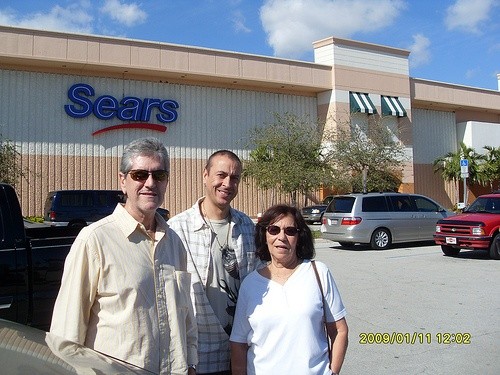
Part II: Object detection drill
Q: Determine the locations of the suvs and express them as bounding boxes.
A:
[43,189,169,227]
[302,195,335,225]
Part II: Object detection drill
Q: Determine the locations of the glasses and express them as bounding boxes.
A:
[129,169,169,181]
[267,225,300,236]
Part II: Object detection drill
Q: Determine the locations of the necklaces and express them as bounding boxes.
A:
[203,204,230,248]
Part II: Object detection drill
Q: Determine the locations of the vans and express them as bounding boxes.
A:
[320,191,459,250]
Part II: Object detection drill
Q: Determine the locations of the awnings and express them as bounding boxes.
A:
[381,96,407,118]
[350,93,377,115]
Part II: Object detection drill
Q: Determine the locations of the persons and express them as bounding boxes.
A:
[229,204,349,375]
[167,150,269,375]
[50,136,200,375]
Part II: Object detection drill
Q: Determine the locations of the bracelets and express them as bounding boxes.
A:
[188,364,196,371]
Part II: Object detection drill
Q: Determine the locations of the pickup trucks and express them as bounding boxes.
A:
[432,193,500,260]
[1,181,81,330]
